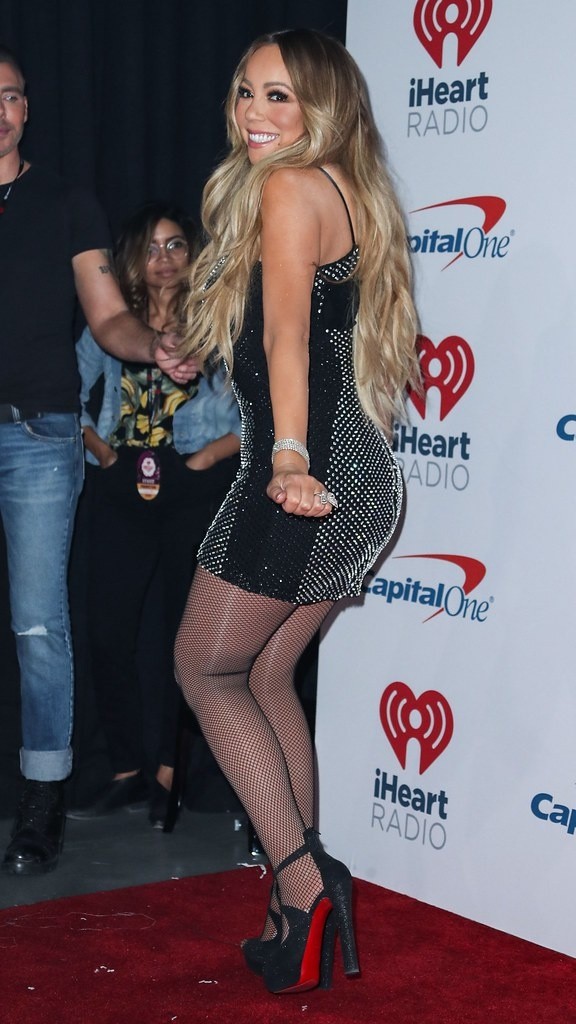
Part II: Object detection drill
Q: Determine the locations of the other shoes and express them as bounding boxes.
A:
[148,774,179,833]
[63,769,152,820]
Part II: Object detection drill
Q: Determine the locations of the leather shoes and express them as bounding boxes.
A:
[2,768,67,875]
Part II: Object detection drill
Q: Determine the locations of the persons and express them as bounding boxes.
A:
[154,26,430,995]
[75,206,246,831]
[0,54,200,878]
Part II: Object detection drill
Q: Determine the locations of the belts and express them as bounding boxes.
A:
[0,401,43,422]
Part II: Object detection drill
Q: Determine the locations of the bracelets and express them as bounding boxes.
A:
[271,439,311,469]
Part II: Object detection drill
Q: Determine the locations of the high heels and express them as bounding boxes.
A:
[261,827,360,993]
[240,876,335,990]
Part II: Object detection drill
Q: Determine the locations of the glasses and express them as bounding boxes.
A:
[145,237,189,265]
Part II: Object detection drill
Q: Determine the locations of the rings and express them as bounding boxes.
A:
[327,491,339,508]
[315,490,327,504]
